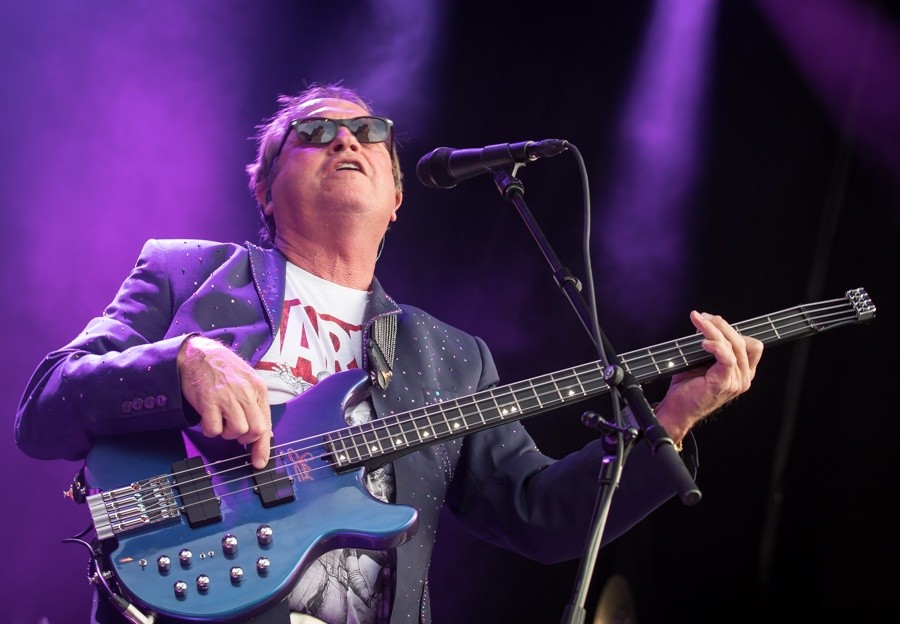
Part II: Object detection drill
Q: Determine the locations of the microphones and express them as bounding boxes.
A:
[416,139,570,188]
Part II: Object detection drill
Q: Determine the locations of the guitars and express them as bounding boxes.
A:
[74,286,877,624]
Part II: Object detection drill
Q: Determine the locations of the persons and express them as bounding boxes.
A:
[14,85,764,624]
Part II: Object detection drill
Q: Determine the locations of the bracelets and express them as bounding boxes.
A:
[651,407,683,451]
